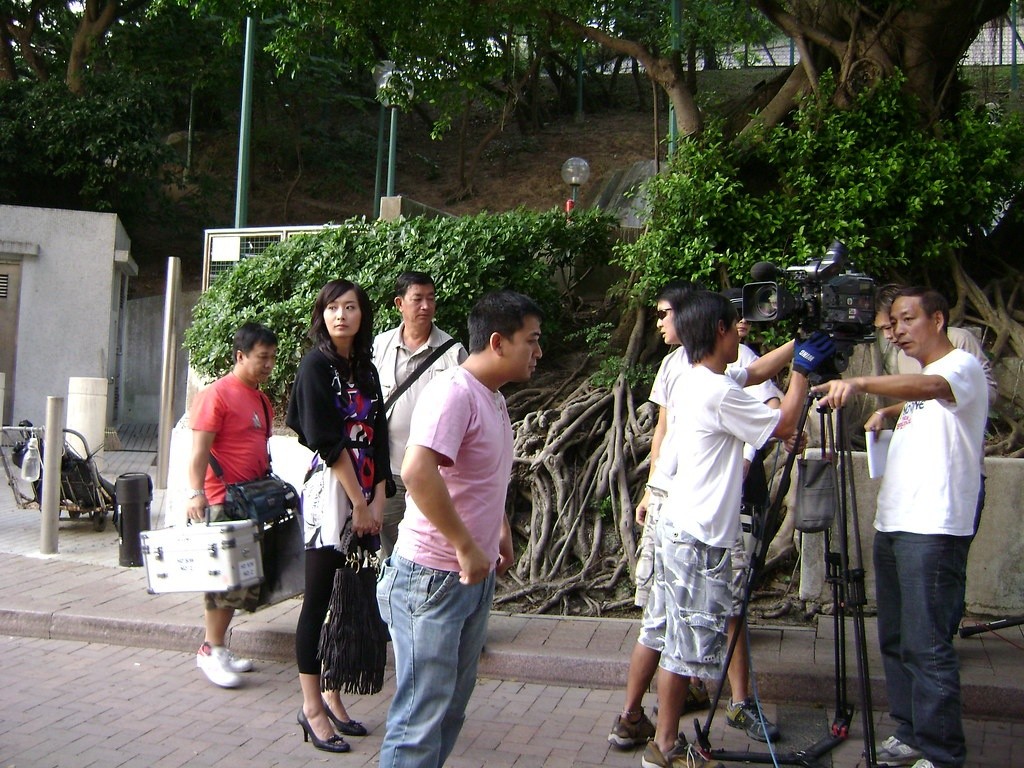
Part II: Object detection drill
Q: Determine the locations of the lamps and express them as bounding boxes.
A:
[560,157,590,207]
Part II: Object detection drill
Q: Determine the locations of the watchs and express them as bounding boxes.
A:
[187,488,205,499]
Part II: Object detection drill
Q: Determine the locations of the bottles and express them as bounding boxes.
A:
[22,439,40,482]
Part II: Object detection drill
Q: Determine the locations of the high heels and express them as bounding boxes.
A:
[297,706,351,753]
[327,707,368,735]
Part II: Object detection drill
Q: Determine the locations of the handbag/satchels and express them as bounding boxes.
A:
[797,459,836,533]
[226,475,302,543]
[318,528,387,695]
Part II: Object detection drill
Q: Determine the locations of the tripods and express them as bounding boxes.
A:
[694,330,890,768]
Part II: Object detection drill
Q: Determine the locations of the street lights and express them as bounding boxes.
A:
[371,60,398,218]
[562,157,592,210]
[376,70,416,195]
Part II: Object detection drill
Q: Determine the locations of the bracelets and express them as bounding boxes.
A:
[875,411,886,420]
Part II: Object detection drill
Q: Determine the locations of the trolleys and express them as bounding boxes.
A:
[0,426,110,533]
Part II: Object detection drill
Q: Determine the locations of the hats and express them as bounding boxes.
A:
[721,287,743,308]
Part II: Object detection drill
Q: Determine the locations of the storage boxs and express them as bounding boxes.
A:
[139,506,266,595]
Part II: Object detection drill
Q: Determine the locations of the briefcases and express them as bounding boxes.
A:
[140,508,267,593]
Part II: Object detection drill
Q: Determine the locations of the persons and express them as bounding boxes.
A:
[811,285,989,768]
[635,279,780,743]
[375,293,544,768]
[369,272,469,559]
[286,278,397,753]
[187,322,279,687]
[874,285,999,633]
[719,288,775,602]
[608,290,837,768]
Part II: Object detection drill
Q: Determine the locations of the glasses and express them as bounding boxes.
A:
[656,309,673,319]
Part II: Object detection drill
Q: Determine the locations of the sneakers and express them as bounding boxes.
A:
[863,736,924,766]
[196,644,240,687]
[654,681,711,715]
[221,647,253,672]
[911,759,935,768]
[609,706,656,747]
[725,695,779,741]
[641,732,724,768]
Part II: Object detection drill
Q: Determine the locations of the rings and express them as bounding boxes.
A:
[375,527,378,531]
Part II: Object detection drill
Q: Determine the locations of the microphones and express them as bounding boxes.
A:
[749,261,790,282]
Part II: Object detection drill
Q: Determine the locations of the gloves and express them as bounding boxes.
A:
[792,330,837,377]
